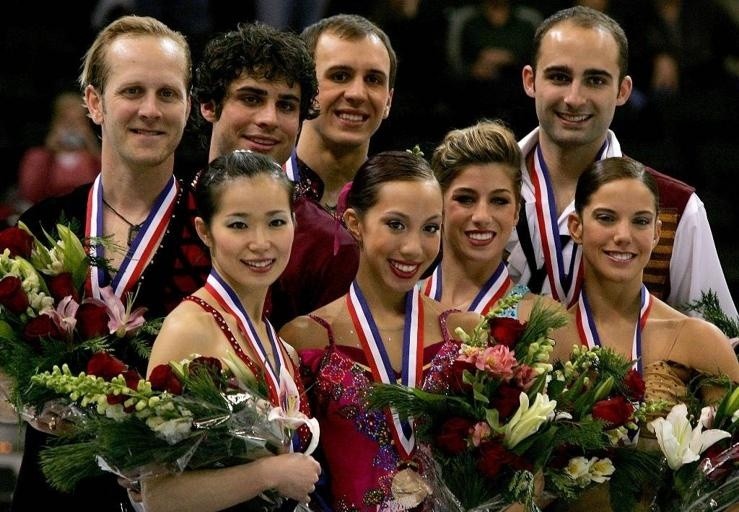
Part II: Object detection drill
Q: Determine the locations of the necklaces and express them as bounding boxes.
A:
[104,197,156,248]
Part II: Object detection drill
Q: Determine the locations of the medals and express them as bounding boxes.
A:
[392,466,429,508]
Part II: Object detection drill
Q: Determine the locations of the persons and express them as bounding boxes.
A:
[278,152,495,512]
[1,14,224,509]
[135,150,321,511]
[419,117,612,511]
[503,6,738,343]
[190,15,359,338]
[1,0,738,186]
[564,157,738,512]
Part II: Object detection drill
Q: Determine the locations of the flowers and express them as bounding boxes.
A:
[0,200,162,433]
[27,355,319,512]
